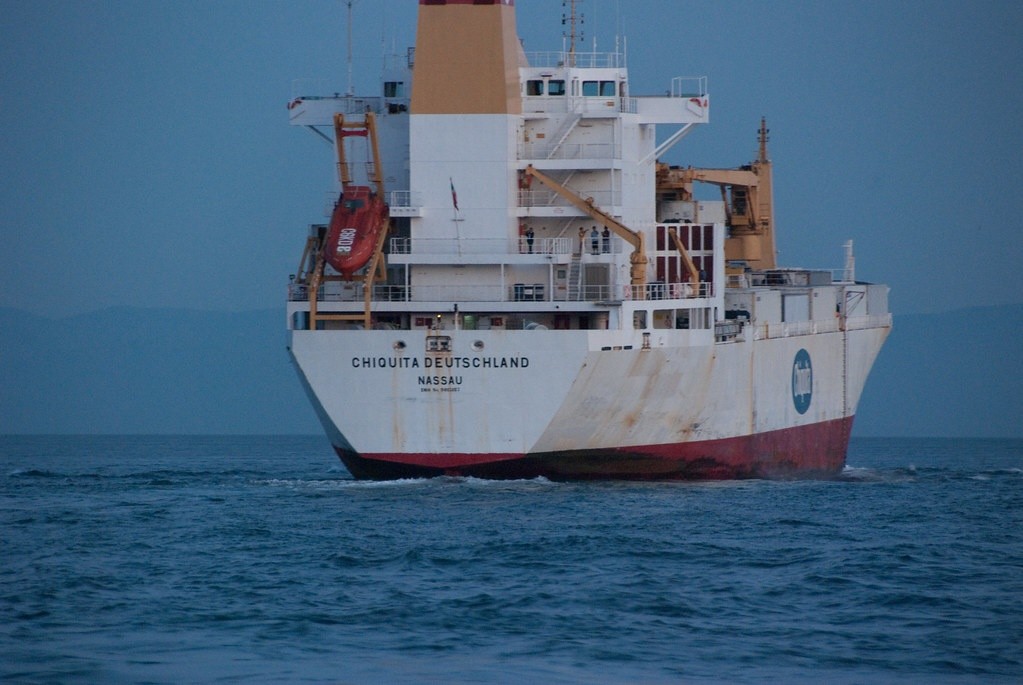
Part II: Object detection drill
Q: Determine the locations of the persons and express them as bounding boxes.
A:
[526,227,534,253]
[578,227,589,252]
[591,226,599,255]
[601,226,610,253]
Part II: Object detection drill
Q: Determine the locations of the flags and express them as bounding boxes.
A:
[450,178,459,211]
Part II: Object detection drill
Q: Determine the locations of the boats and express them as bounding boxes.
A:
[280,0,897,480]
